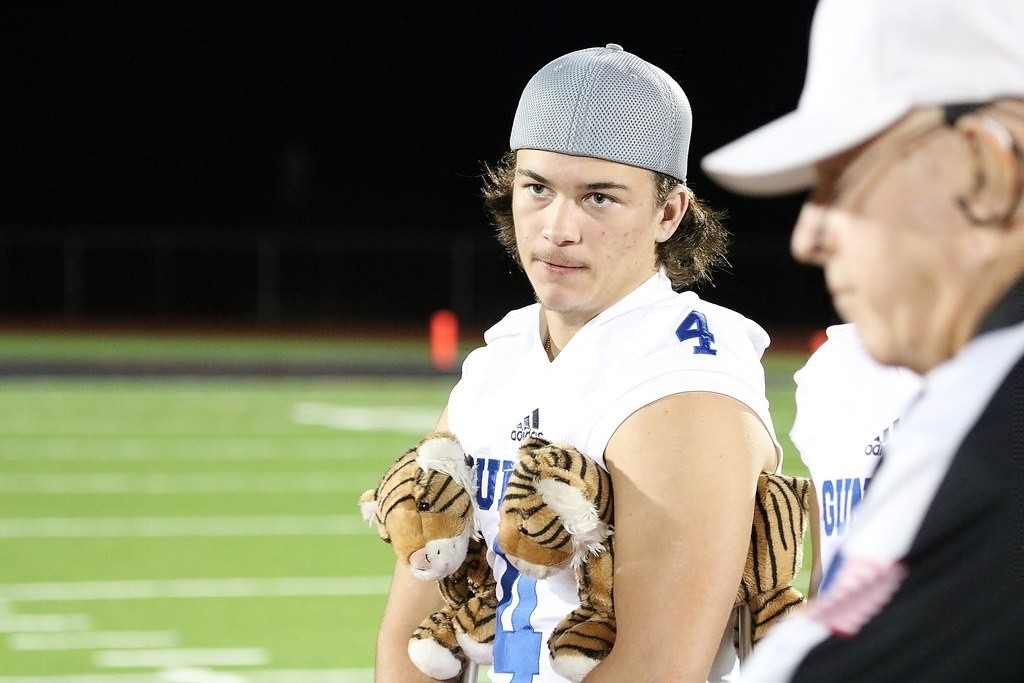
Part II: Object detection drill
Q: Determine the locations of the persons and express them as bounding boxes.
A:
[375,44,784,683]
[700,1,1024,683]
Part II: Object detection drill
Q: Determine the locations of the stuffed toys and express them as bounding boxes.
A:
[498,437,811,683]
[361,432,498,680]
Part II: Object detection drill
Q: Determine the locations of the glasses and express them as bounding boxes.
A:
[815,119,955,210]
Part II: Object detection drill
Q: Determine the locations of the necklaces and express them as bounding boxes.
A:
[545,332,552,354]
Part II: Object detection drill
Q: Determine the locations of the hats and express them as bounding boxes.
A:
[510,43,692,182]
[699,0,1024,196]
